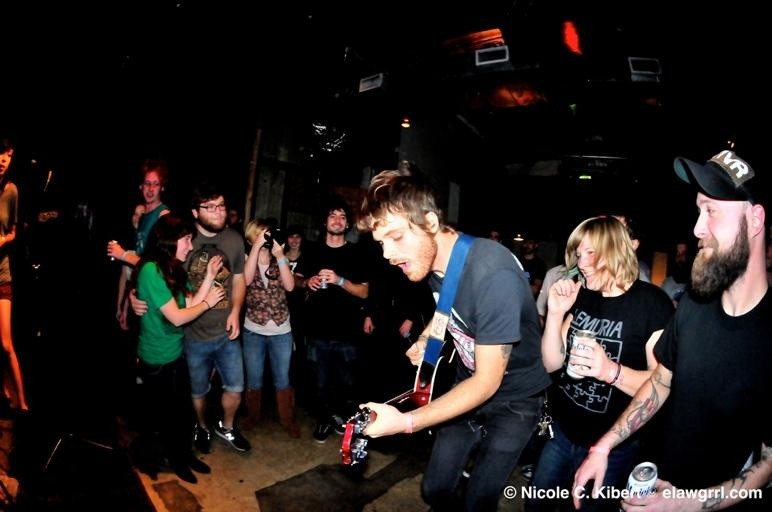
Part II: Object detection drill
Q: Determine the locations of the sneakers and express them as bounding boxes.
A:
[172,389,346,483]
[520,464,533,481]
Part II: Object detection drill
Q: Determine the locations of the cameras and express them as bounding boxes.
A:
[264,228,286,248]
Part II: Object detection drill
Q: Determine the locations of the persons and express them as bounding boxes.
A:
[1,143,692,454]
[524,214,676,511]
[569,140,772,512]
[358,168,549,509]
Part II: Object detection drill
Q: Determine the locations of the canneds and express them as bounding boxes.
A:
[320,278,328,289]
[566,329,595,380]
[626,461,658,498]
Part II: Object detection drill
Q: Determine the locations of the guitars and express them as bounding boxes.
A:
[339,340,458,466]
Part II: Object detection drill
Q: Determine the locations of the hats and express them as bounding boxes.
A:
[673,146,770,201]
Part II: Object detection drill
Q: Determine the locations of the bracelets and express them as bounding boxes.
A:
[602,358,626,385]
[416,334,428,342]
[403,408,416,434]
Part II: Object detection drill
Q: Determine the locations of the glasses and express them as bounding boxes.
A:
[201,203,225,212]
